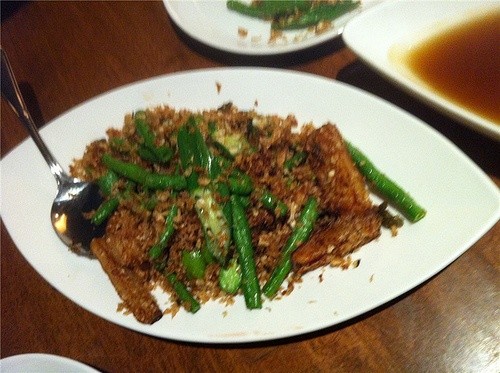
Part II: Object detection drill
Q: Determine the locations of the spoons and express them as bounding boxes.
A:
[1,51,106,260]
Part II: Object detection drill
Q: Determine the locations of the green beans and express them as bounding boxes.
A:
[91,110,427,313]
[227,0,359,30]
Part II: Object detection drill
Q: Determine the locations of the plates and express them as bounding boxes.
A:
[338,3,499,142]
[0,69,500,347]
[0,354,98,373]
[154,1,374,60]
